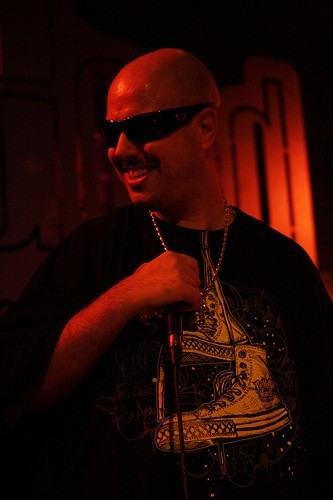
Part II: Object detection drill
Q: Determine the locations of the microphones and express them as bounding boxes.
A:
[130,222,185,366]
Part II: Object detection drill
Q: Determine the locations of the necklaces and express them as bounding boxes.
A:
[147,198,231,297]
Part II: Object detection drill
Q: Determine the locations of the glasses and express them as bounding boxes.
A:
[100,104,213,146]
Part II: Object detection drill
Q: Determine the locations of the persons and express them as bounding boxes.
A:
[1,47,333,500]
